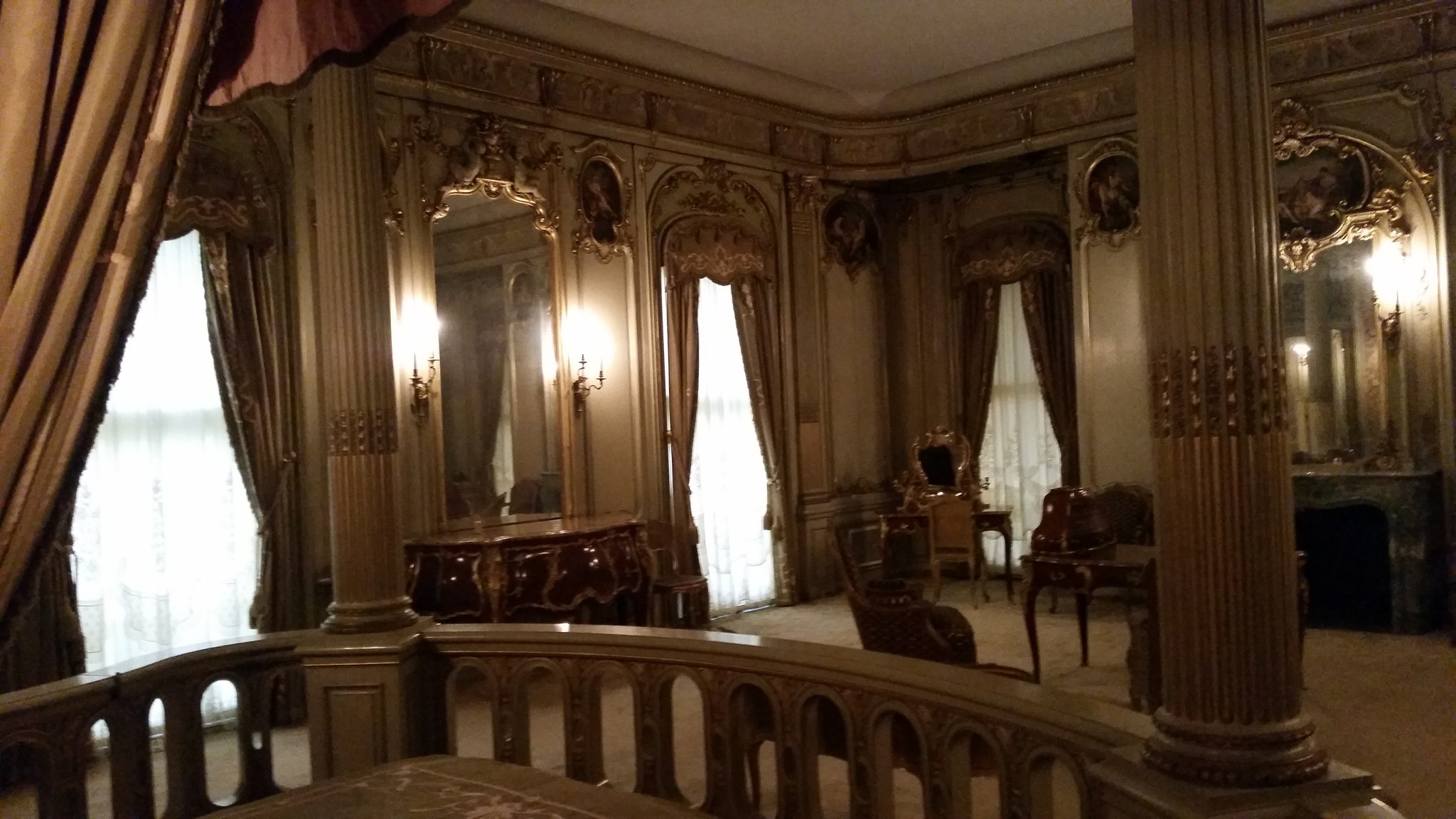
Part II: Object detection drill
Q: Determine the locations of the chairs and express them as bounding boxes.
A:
[927,500,991,610]
[1048,481,1155,615]
[837,555,1031,681]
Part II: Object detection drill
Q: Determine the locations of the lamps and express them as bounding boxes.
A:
[1367,253,1404,356]
[400,315,440,426]
[566,312,608,418]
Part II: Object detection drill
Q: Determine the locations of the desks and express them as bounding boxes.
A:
[1017,543,1309,712]
[880,504,1018,608]
[403,514,659,627]
[1290,467,1443,633]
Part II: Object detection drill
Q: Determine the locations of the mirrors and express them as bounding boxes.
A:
[911,427,969,497]
[1273,211,1391,462]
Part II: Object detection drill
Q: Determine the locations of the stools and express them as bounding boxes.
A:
[653,574,710,631]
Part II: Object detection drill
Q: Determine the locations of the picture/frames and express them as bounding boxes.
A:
[566,138,639,264]
[1272,140,1371,239]
[822,185,884,283]
[1072,136,1144,256]
